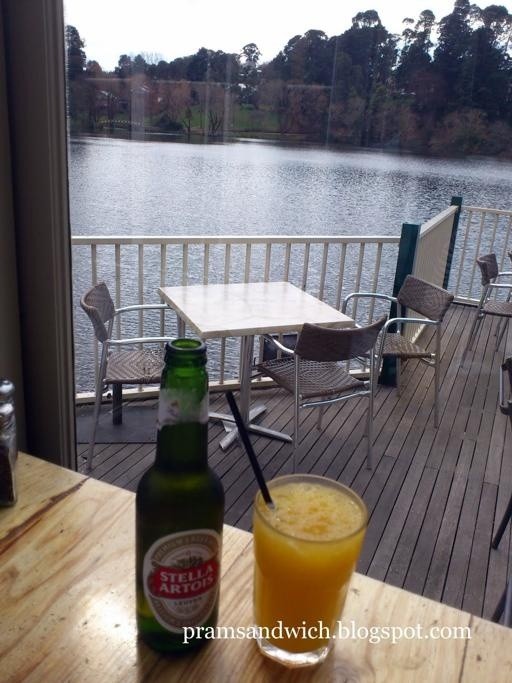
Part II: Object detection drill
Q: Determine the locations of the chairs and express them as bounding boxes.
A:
[459,251,512,368]
[80,280,182,470]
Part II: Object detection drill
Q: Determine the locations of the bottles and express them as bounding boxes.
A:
[135,337,225,655]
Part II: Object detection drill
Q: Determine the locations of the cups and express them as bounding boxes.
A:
[252,473,368,669]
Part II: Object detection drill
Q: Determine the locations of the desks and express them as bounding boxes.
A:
[156,279,357,452]
[1,451,511,683]
